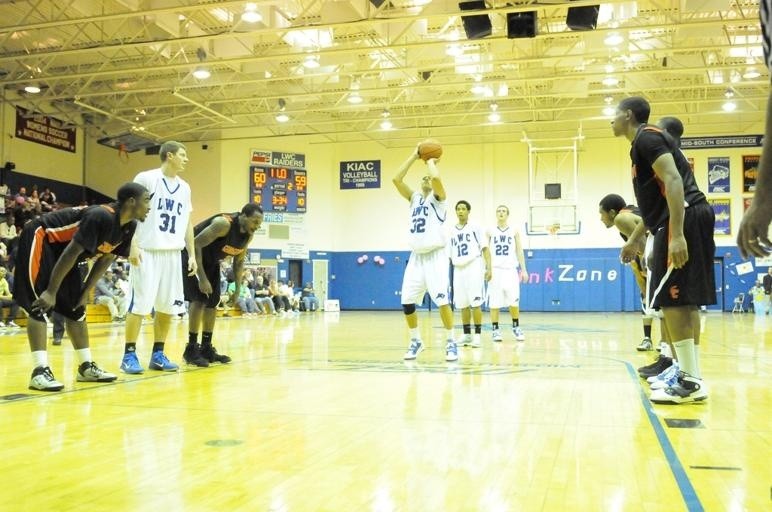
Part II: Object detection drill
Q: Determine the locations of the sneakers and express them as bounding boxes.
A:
[404,338,424,359]
[464,334,480,348]
[119,352,144,374]
[512,327,525,341]
[637,337,708,405]
[149,351,179,371]
[29,366,64,392]
[445,339,459,360]
[0,321,6,327]
[241,308,321,316]
[76,362,118,381]
[183,343,231,367]
[493,329,503,341]
[7,320,17,326]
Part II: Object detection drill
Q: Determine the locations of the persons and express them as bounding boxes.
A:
[487,206,527,343]
[599,95,714,404]
[748,280,766,316]
[11,182,152,391]
[214,253,324,318]
[120,141,198,375]
[0,184,191,330]
[762,266,772,316]
[391,143,460,362]
[182,202,263,368]
[448,201,493,347]
[735,0,772,260]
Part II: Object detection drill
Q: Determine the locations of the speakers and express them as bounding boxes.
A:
[459,1,493,38]
[507,1,538,38]
[566,0,599,29]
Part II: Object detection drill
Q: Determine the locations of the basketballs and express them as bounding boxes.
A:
[419,138,442,162]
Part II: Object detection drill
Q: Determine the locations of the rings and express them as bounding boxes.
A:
[748,239,757,243]
